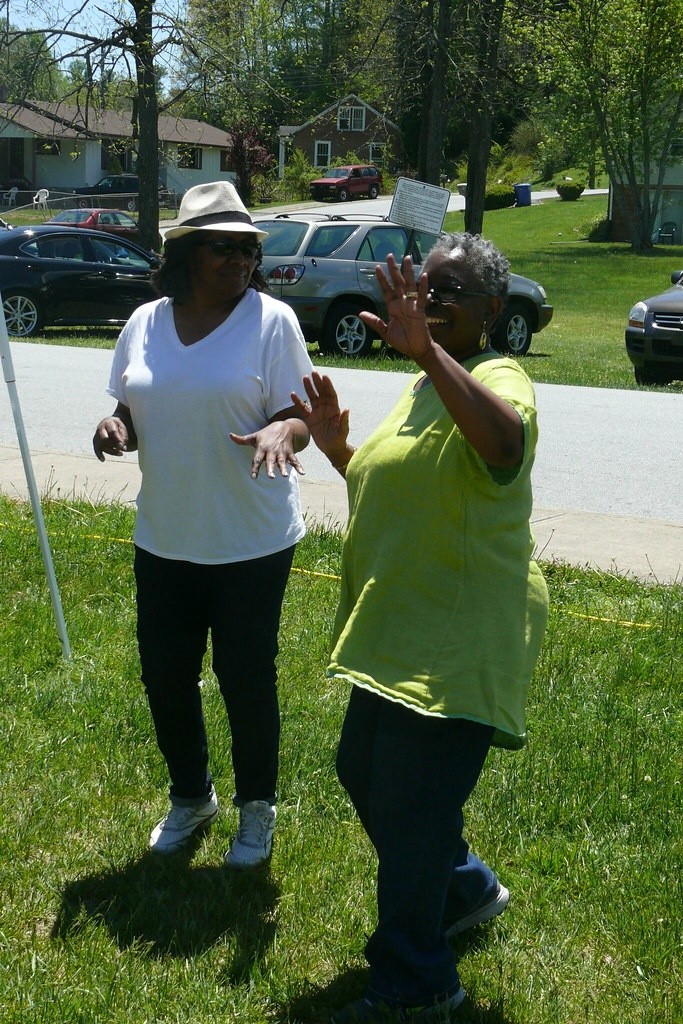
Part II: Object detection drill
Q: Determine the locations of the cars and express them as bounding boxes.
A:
[0,209,165,337]
[625,272,683,386]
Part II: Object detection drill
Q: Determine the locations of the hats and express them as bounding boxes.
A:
[165,181,269,245]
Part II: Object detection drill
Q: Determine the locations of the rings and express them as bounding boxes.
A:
[406,292,419,297]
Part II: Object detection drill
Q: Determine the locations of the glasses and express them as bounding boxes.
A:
[417,283,490,303]
[185,237,260,257]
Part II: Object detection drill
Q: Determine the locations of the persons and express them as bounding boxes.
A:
[92,179,313,863]
[290,229,548,1016]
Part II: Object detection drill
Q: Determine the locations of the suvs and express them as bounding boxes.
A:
[72,173,170,212]
[310,166,383,202]
[251,213,554,357]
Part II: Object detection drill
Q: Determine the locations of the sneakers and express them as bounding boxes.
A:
[226,799,278,868]
[150,785,218,852]
[330,972,464,1024]
[441,878,509,938]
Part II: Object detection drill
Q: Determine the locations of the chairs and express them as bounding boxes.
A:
[657,221,677,243]
[374,243,402,263]
[2,187,18,208]
[32,189,49,210]
[102,217,110,224]
[63,242,75,260]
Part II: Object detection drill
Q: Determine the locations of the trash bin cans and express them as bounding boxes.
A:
[514,183,531,206]
